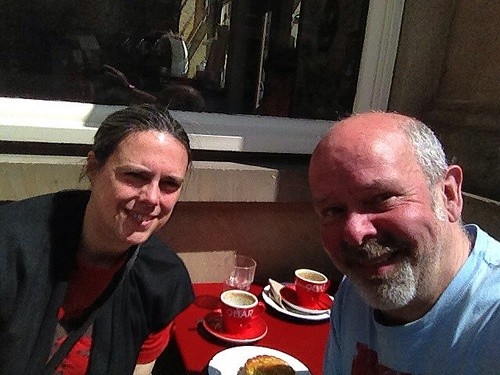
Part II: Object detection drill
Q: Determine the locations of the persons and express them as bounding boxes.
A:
[307,111,500,375]
[0,102,194,374]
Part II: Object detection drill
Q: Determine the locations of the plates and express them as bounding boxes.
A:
[207,344,312,375]
[261,282,336,321]
[202,309,268,345]
[279,282,335,314]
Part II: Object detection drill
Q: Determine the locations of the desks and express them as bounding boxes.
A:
[173,282,333,375]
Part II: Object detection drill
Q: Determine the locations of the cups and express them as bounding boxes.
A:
[223,254,257,295]
[294,268,328,307]
[220,289,266,335]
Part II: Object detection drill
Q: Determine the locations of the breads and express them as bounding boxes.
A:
[245,355,296,375]
